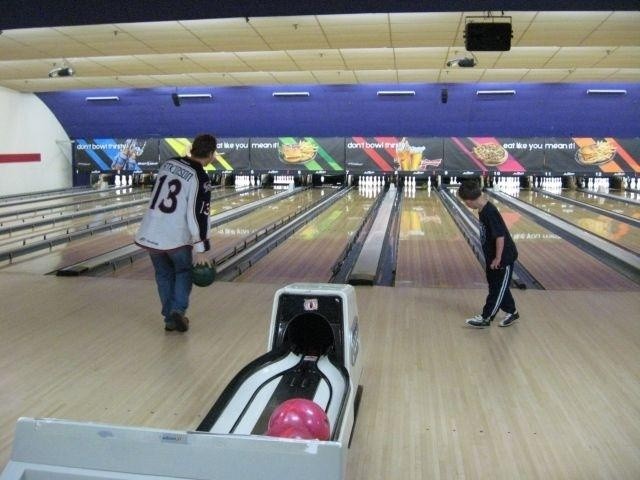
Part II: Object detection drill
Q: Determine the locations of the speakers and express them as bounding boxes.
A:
[172,93,179,106]
[442,89,447,103]
[465,23,511,51]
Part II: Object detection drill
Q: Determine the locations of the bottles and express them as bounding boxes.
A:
[113,147,137,172]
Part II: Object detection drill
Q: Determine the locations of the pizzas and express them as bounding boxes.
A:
[472,143,506,164]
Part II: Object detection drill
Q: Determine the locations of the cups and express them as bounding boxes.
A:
[395,146,424,171]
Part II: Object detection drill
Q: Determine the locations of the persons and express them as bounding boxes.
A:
[133,134,217,332]
[458,179,519,328]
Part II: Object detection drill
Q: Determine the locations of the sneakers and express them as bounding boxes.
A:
[497,310,520,328]
[164,313,189,332]
[465,315,491,327]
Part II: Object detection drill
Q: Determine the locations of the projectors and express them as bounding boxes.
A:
[48,66,73,77]
[447,58,474,67]
